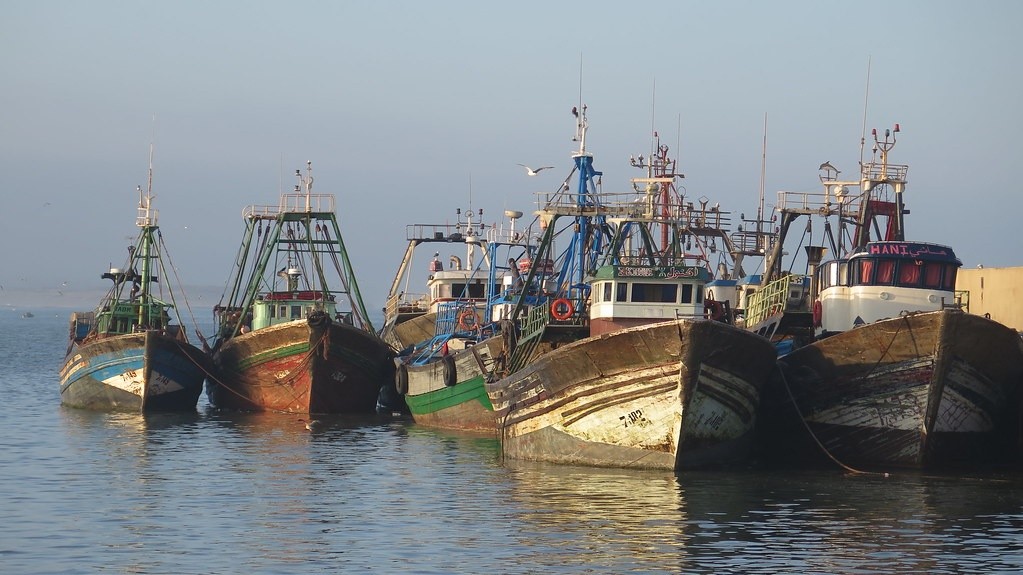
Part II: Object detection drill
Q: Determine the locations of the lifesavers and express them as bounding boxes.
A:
[812,301,821,327]
[231,312,248,329]
[395,364,408,394]
[705,300,723,320]
[460,309,479,331]
[552,298,573,319]
[443,355,456,386]
[306,310,332,330]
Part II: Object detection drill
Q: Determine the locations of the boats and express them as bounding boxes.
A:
[380,51,1023,475]
[57,117,212,413]
[205,146,397,418]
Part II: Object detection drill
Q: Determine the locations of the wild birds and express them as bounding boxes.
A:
[517,164,554,176]
[564,182,569,192]
